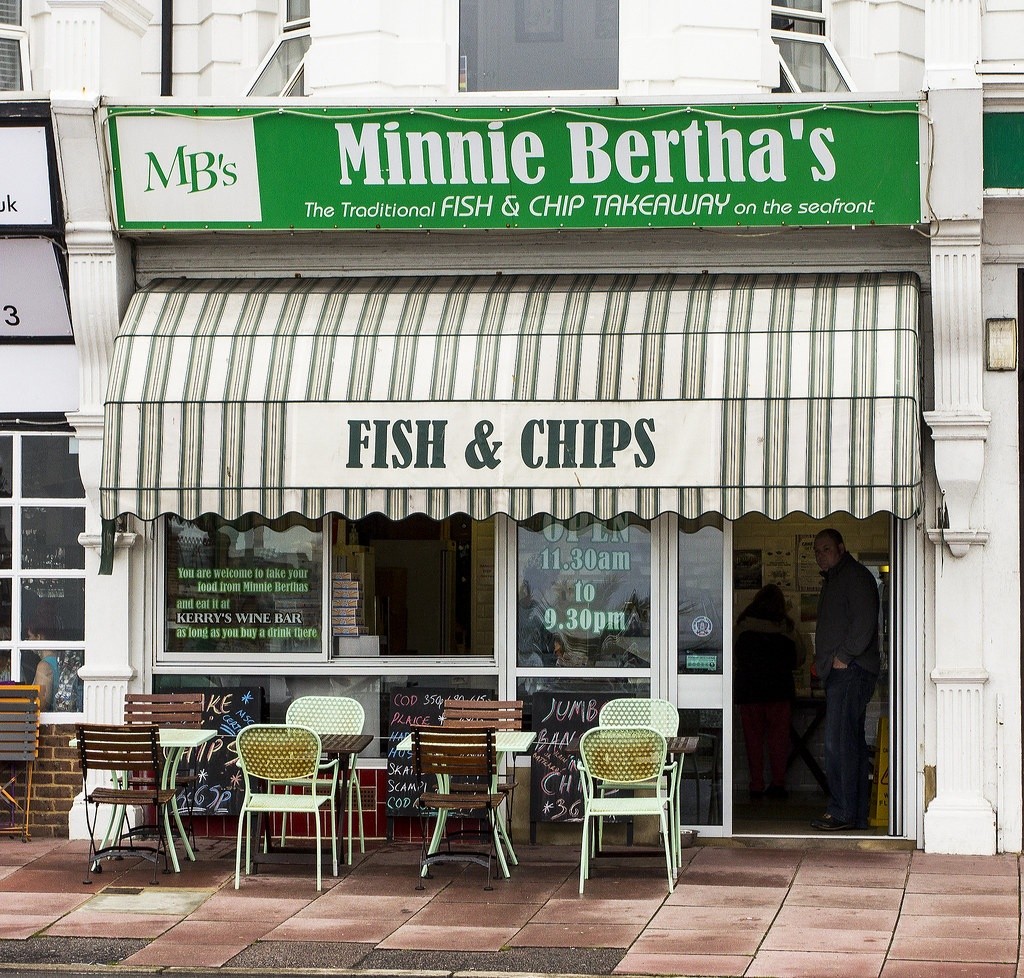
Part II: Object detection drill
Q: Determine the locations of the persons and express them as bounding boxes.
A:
[808,528,880,832]
[0,649,26,685]
[28,618,81,710]
[732,584,808,792]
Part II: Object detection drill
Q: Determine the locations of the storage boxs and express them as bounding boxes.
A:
[332,572,369,636]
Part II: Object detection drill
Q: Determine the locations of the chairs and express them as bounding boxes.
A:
[577,699,684,894]
[410,699,523,891]
[235,696,365,891]
[0,682,41,842]
[74,693,202,884]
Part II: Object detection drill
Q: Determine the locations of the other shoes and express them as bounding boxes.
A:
[749,790,768,802]
[810,810,868,831]
[766,782,792,796]
[814,795,833,812]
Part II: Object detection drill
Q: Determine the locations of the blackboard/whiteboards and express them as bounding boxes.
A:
[156,686,266,817]
[389,686,495,818]
[530,691,634,823]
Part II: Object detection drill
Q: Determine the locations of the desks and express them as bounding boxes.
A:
[774,696,827,791]
[564,736,700,881]
[396,731,537,878]
[251,734,374,875]
[69,728,218,873]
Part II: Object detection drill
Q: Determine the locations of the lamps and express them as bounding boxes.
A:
[986,315,1017,372]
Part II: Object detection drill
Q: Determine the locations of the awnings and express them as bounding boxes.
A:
[97,276,930,520]
